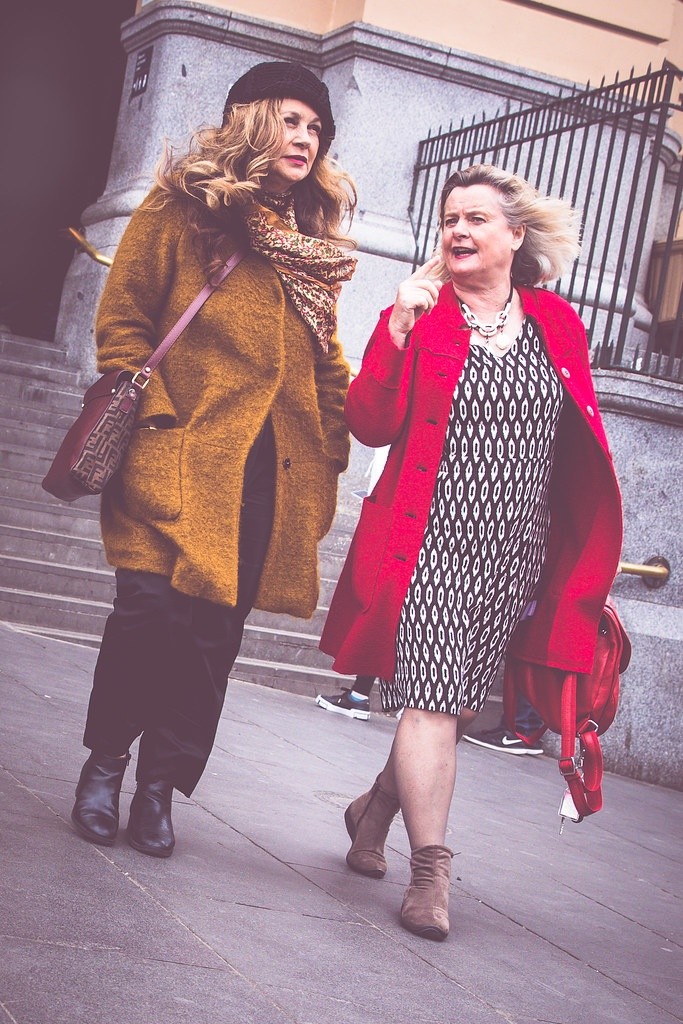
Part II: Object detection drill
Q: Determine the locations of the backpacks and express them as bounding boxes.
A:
[502,591,632,818]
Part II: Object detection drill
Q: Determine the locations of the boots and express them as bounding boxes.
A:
[70,750,131,846]
[127,763,175,858]
[342,771,401,879]
[400,843,463,940]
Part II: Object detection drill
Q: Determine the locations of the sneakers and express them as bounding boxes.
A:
[316,685,371,720]
[464,725,544,755]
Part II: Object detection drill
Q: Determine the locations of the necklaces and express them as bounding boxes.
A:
[455,277,514,350]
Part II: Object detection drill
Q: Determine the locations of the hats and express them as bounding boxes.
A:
[224,61,337,159]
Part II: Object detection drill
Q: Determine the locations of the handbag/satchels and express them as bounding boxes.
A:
[43,370,142,501]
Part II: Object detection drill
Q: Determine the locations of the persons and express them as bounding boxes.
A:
[343,165,624,942]
[71,62,351,858]
[314,675,376,721]
[461,687,544,755]
